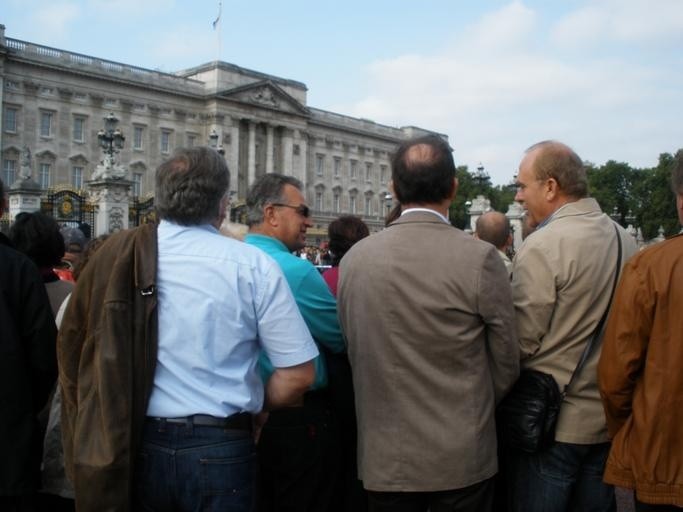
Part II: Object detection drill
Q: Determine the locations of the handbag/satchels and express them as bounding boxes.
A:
[492,366,565,460]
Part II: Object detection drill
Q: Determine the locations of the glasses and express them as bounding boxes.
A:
[270,199,310,219]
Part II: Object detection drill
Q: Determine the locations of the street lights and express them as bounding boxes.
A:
[471,162,491,197]
[204,128,225,157]
[463,198,471,230]
[382,190,391,224]
[624,208,636,226]
[87,112,132,243]
[609,205,622,224]
[511,172,519,200]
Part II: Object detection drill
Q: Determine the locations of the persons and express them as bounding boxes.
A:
[12,210,546,330]
[597,146,682,512]
[54,143,321,512]
[239,172,345,512]
[509,138,642,512]
[334,127,520,511]
[0,175,60,512]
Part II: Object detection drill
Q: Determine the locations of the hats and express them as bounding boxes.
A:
[59,224,86,254]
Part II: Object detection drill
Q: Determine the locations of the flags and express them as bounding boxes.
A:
[211,8,221,30]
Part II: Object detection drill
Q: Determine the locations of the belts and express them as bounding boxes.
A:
[144,414,257,431]
